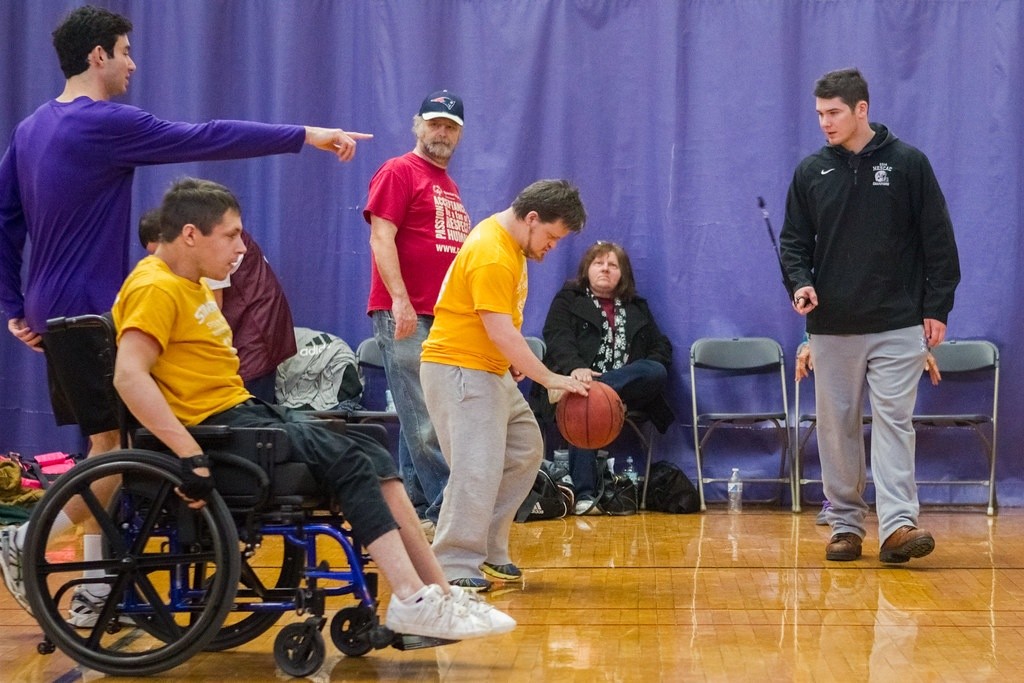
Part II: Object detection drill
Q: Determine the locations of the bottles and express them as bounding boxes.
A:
[621,456,638,486]
[727,468,742,514]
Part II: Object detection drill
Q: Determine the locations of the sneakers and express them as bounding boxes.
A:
[69,585,137,627]
[0,525,34,617]
[879,525,935,563]
[450,577,491,591]
[479,562,521,580]
[825,533,862,561]
[385,584,491,640]
[450,588,516,634]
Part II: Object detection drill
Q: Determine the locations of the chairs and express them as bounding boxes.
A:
[356,337,1001,514]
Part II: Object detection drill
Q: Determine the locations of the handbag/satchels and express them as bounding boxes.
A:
[572,460,637,516]
[647,460,700,514]
[513,459,575,523]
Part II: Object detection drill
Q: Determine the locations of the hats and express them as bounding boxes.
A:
[419,90,464,126]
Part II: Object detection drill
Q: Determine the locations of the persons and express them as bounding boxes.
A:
[416,178,592,594]
[136,206,299,402]
[535,237,673,515]
[0,5,375,625]
[109,181,518,641]
[363,89,473,539]
[777,69,963,565]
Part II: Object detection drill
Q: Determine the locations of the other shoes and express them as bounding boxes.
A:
[547,390,564,405]
[571,499,602,516]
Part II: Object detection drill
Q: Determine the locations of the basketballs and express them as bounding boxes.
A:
[556,379,625,449]
